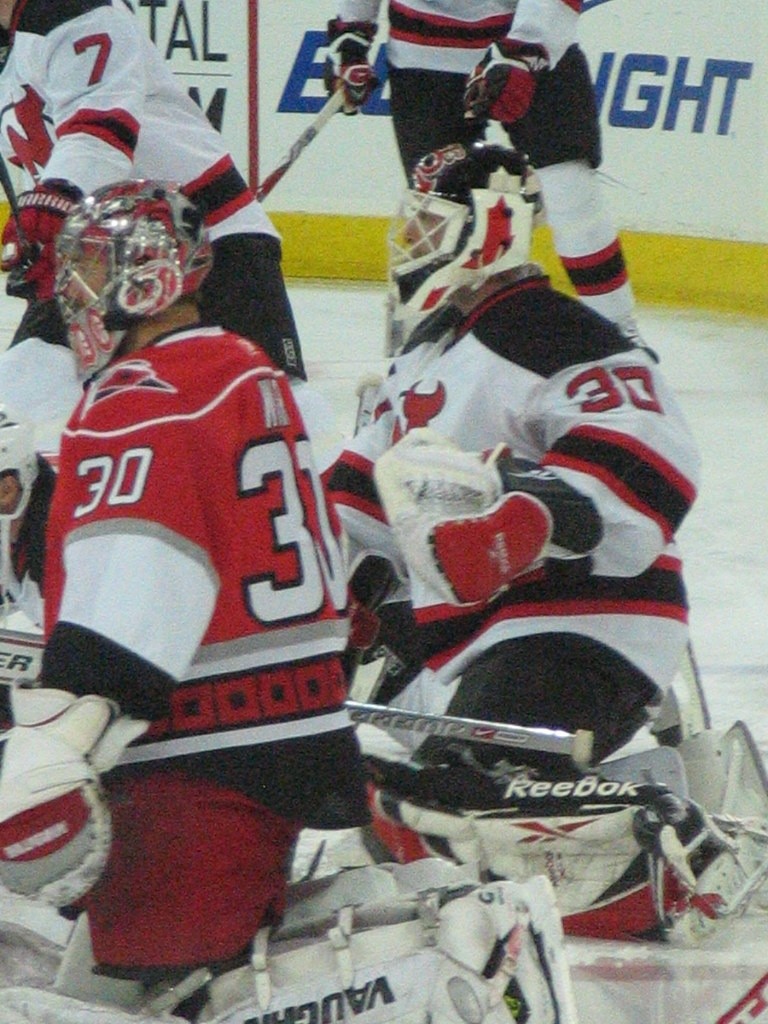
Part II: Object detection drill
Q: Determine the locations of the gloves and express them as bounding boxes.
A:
[323,16,377,116]
[461,37,550,127]
[0,179,85,302]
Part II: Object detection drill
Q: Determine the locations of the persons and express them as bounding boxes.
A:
[319,145,768,944]
[0,0,311,383]
[0,180,574,1024]
[324,0,645,346]
[0,403,60,630]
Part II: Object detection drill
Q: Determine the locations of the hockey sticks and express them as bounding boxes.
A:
[352,373,381,436]
[0,628,592,765]
[257,84,347,202]
[1,154,36,305]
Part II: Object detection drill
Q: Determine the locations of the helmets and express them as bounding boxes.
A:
[0,404,39,489]
[396,142,548,303]
[52,178,216,381]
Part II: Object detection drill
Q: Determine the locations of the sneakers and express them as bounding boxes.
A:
[442,869,577,1024]
[680,719,766,906]
